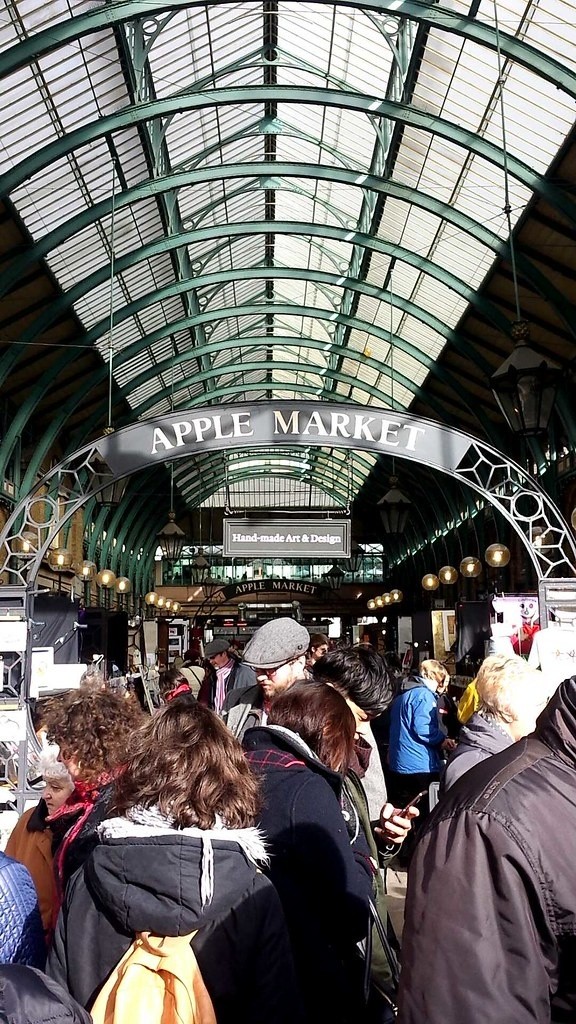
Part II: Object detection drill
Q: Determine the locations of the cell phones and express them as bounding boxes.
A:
[381,790,428,834]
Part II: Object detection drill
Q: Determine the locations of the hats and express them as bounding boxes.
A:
[203,638,230,660]
[240,617,311,670]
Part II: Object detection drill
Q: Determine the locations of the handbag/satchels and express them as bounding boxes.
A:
[356,894,403,1024]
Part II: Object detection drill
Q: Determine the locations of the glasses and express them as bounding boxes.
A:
[208,653,221,659]
[249,657,300,676]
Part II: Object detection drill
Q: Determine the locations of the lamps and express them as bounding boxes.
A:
[75,559,131,596]
[146,590,181,613]
[11,531,38,561]
[341,540,366,572]
[76,157,127,505]
[326,558,343,591]
[183,455,208,587]
[28,618,48,642]
[488,1,559,442]
[457,557,482,579]
[438,564,457,587]
[363,587,405,610]
[159,338,189,576]
[377,457,413,534]
[48,547,73,574]
[485,541,511,569]
[319,576,331,600]
[201,497,217,597]
[419,573,438,594]
[525,509,576,559]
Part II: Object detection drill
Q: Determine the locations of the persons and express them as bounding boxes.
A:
[387,639,550,799]
[397,675,576,1024]
[43,691,301,1024]
[239,644,420,1024]
[3,686,157,952]
[143,617,395,746]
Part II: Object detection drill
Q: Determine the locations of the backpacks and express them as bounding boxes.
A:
[395,743,480,869]
[85,929,219,1024]
